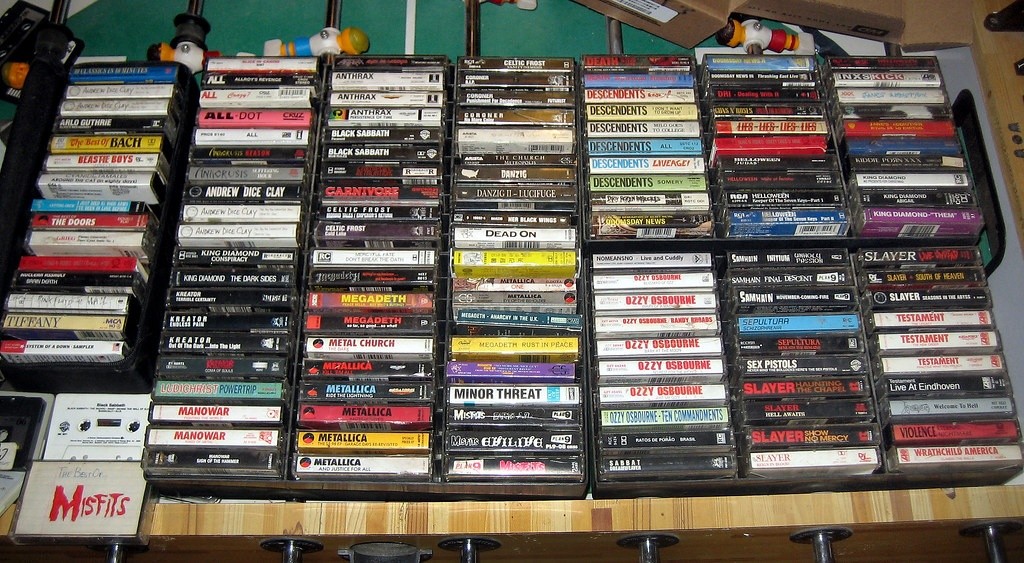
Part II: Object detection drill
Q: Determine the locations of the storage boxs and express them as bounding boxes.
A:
[574,0,729,50]
[728,0,905,44]
[901,0,974,54]
